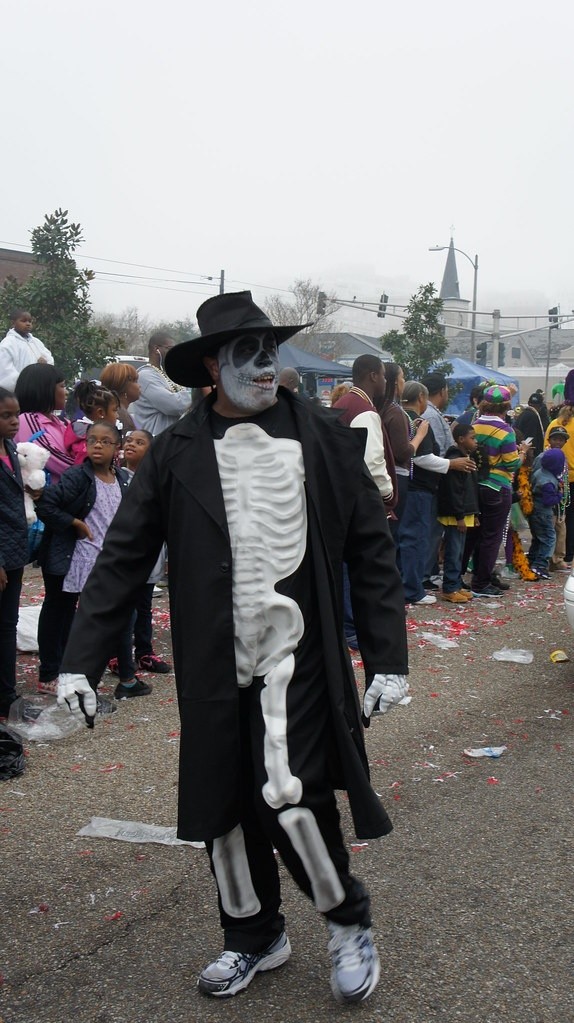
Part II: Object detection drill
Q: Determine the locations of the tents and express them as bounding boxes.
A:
[424,358,519,414]
[280,345,352,383]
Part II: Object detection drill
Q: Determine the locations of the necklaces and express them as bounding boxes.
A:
[558,475,570,522]
[349,387,373,407]
[404,411,413,480]
[503,509,512,546]
[152,365,176,393]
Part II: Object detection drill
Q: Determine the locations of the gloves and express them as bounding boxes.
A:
[57,671,97,730]
[360,673,410,728]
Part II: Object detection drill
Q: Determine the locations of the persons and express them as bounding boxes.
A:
[0,387,40,722]
[528,448,565,578]
[377,363,550,605]
[278,367,320,407]
[40,420,153,713]
[59,333,193,714]
[14,363,80,693]
[332,354,399,652]
[57,291,408,1006]
[543,425,571,571]
[544,369,574,565]
[0,310,55,393]
[110,429,171,674]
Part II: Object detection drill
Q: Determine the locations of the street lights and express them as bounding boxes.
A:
[427,243,479,363]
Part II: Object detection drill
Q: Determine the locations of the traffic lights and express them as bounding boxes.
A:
[498,342,506,367]
[476,342,488,366]
[315,291,327,315]
[548,307,559,328]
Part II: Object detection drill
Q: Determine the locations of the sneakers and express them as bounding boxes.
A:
[95,693,118,714]
[107,658,121,675]
[465,559,473,573]
[135,654,170,673]
[197,930,292,998]
[441,591,468,604]
[458,586,474,600]
[471,583,504,599]
[530,566,552,580]
[491,571,509,590]
[326,920,381,1004]
[115,676,153,700]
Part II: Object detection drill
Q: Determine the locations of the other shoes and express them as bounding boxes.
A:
[36,676,58,695]
[2,695,41,719]
[152,585,166,597]
[554,561,572,573]
[421,579,439,591]
[157,575,168,586]
[431,579,443,589]
[413,594,437,604]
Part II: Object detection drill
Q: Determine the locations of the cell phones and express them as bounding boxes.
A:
[416,419,423,428]
[525,437,534,444]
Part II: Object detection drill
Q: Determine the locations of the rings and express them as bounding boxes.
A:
[466,466,468,469]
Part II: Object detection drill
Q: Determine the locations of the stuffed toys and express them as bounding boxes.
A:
[16,442,50,523]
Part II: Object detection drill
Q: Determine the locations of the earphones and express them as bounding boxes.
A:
[157,349,160,354]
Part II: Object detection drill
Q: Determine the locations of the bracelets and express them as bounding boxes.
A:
[519,451,527,459]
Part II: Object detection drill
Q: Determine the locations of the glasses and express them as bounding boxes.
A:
[86,436,118,447]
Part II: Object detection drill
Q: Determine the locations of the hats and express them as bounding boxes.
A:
[162,290,313,387]
[529,393,544,403]
[549,427,569,440]
[483,386,512,403]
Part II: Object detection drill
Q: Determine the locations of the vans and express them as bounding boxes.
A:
[74,354,150,380]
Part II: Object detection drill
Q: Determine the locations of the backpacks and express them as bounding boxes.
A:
[470,425,508,477]
